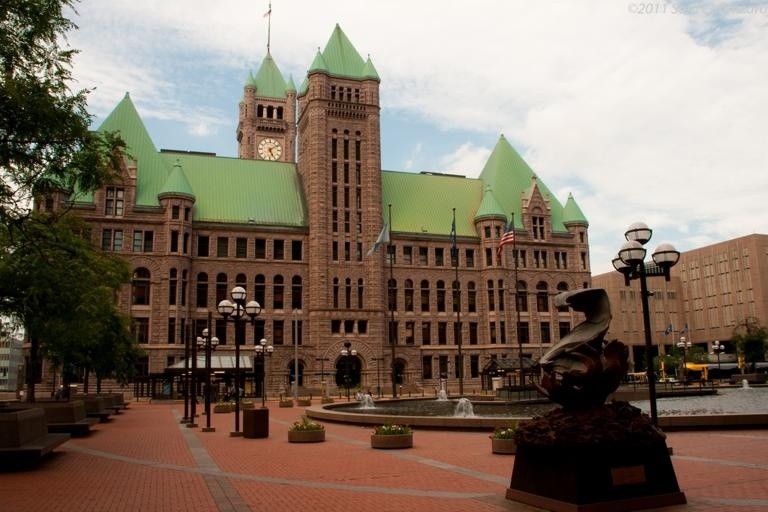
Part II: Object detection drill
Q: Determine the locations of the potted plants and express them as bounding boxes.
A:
[368,419,414,450]
[489,427,517,455]
[214,395,333,413]
[286,416,326,443]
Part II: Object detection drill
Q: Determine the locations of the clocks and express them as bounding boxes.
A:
[256,136,283,161]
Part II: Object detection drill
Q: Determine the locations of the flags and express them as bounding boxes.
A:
[450,221,455,255]
[664,324,672,336]
[680,323,688,335]
[495,220,514,257]
[367,220,390,256]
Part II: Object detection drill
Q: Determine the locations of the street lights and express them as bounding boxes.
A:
[711,339,725,382]
[339,341,355,402]
[609,220,682,434]
[217,285,260,434]
[196,328,221,416]
[676,335,690,384]
[253,339,273,409]
